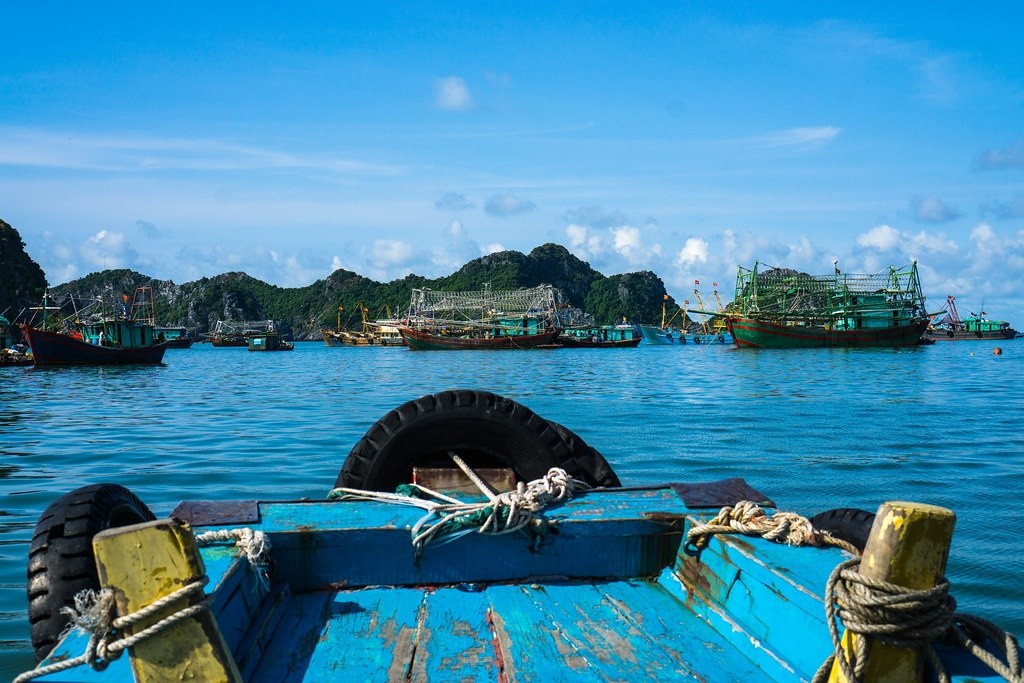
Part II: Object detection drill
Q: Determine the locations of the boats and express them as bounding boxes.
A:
[321,304,407,346]
[686,258,949,349]
[396,279,564,350]
[640,289,735,346]
[0,286,196,367]
[921,293,1018,340]
[199,317,295,350]
[562,337,644,348]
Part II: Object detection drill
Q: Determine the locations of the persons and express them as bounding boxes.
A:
[632,328,639,339]
[404,317,494,341]
[746,312,836,330]
[70,328,84,341]
[621,330,624,340]
[928,325,965,332]
[615,319,654,327]
[521,326,607,343]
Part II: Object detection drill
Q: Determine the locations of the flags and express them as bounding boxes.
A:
[948,296,955,300]
[338,306,368,312]
[664,280,717,304]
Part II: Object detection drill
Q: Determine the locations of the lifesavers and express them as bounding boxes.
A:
[380,339,387,346]
[24,481,160,660]
[809,506,876,554]
[351,338,356,344]
[330,390,619,489]
[368,338,373,344]
